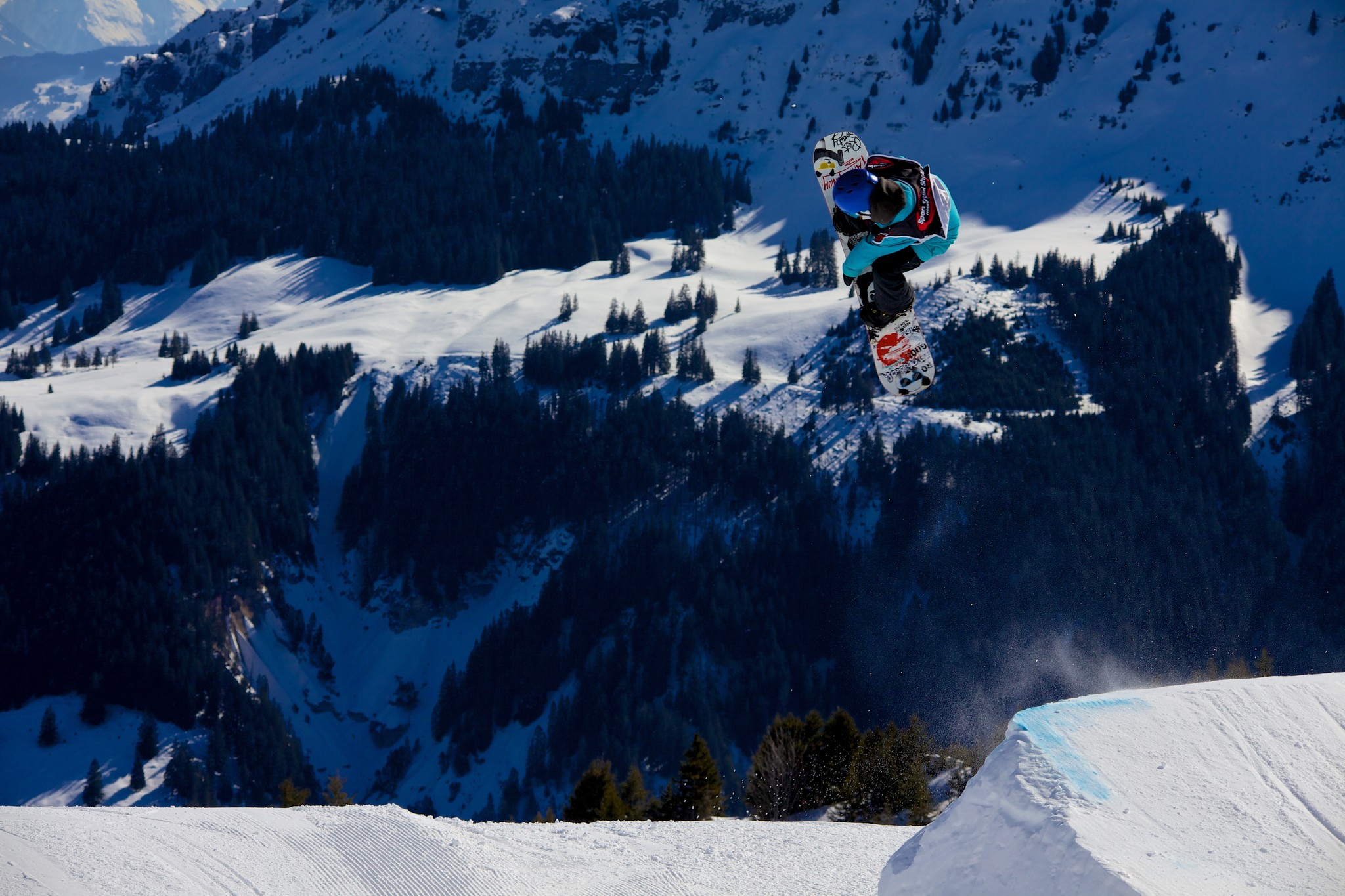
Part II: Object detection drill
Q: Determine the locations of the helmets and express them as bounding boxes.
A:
[833,169,879,218]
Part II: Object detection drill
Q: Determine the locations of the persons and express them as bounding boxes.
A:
[829,146,960,327]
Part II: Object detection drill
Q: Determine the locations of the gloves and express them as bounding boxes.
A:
[843,274,856,286]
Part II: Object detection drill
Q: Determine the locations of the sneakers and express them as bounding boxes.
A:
[867,295,915,326]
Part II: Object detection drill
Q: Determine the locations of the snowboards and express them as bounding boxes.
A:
[813,132,935,396]
